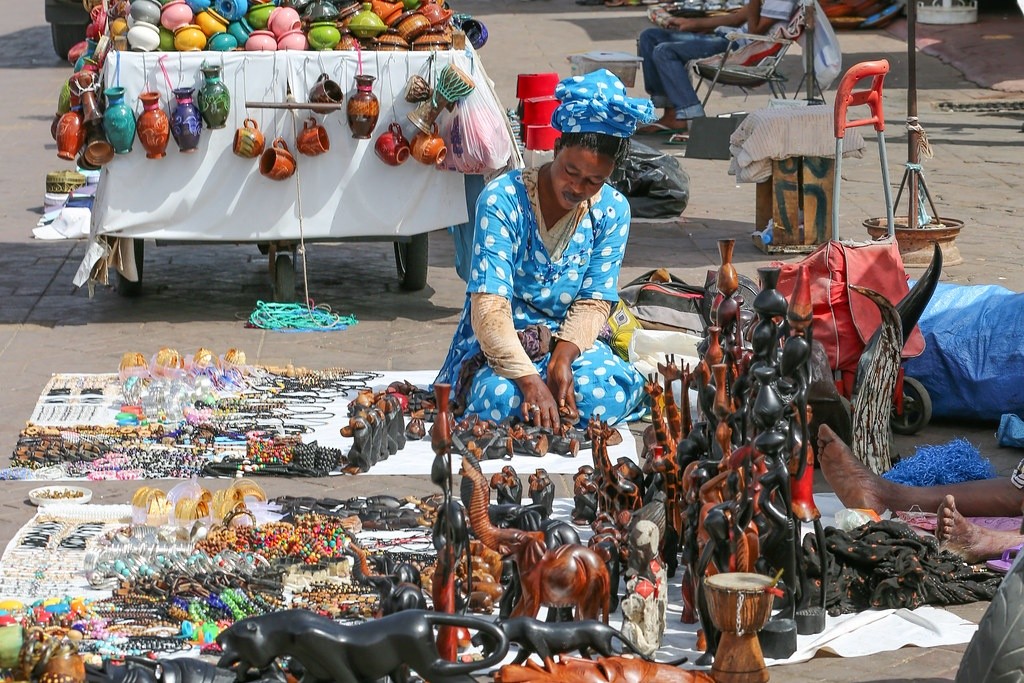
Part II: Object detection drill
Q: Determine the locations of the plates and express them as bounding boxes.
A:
[29,485,93,504]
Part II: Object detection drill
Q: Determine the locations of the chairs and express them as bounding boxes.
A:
[692,5,804,109]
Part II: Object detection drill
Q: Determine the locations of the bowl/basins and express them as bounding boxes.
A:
[129,0,488,52]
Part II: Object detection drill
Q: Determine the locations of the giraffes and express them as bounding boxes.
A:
[644,373,688,549]
[657,353,697,442]
[584,412,642,522]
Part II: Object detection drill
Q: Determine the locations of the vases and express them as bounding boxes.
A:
[102,87,136,154]
[168,87,202,154]
[137,92,170,159]
[197,66,230,130]
[57,112,86,160]
[67,0,339,75]
[347,75,380,140]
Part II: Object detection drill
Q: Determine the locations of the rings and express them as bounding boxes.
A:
[531,405,539,413]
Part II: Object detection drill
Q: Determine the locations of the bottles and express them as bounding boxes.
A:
[347,75,379,139]
[51,57,231,168]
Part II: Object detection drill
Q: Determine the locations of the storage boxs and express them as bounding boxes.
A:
[567,52,643,89]
[754,157,834,256]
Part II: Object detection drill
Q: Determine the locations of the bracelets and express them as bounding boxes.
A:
[0,436,201,479]
[248,435,340,471]
[84,550,351,619]
[133,478,266,528]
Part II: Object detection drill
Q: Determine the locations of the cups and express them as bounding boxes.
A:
[259,137,297,181]
[232,117,266,158]
[374,122,410,166]
[296,117,330,156]
[410,120,447,165]
[308,73,343,115]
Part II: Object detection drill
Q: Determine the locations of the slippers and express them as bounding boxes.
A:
[634,123,687,136]
[662,133,690,145]
[986,543,1024,573]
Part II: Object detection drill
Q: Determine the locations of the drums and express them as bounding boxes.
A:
[703,572,779,682]
[404,75,458,113]
[407,63,476,135]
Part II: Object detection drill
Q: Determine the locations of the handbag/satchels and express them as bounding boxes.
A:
[618,270,707,337]
[803,0,841,91]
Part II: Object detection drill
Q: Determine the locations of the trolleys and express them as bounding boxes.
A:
[795,57,934,469]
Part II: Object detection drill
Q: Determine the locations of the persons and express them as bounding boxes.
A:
[427,68,651,432]
[639,0,798,145]
[818,423,1024,564]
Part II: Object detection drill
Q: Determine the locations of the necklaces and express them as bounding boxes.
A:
[76,593,191,660]
[99,368,380,452]
[520,182,614,285]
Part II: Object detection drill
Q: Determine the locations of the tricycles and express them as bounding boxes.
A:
[97,1,475,300]
